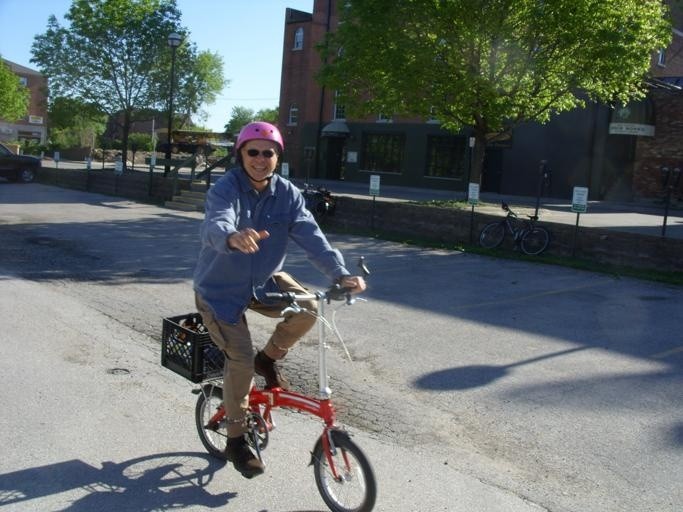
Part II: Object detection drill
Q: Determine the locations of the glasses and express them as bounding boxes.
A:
[243,149,274,158]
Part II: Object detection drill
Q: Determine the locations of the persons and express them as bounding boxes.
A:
[192,121,367,475]
[303,149,312,184]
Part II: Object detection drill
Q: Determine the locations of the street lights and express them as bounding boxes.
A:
[165,31,181,173]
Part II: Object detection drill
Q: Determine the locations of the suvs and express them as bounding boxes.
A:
[0,143,40,184]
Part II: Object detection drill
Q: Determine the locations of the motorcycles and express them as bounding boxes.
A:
[301,183,335,224]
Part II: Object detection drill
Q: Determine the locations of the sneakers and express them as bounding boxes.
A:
[255,346,289,391]
[224,440,266,475]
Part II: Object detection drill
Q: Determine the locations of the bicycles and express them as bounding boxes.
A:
[187,255,377,511]
[479,200,550,256]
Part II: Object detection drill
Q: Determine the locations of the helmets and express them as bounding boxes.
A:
[236,122,285,153]
